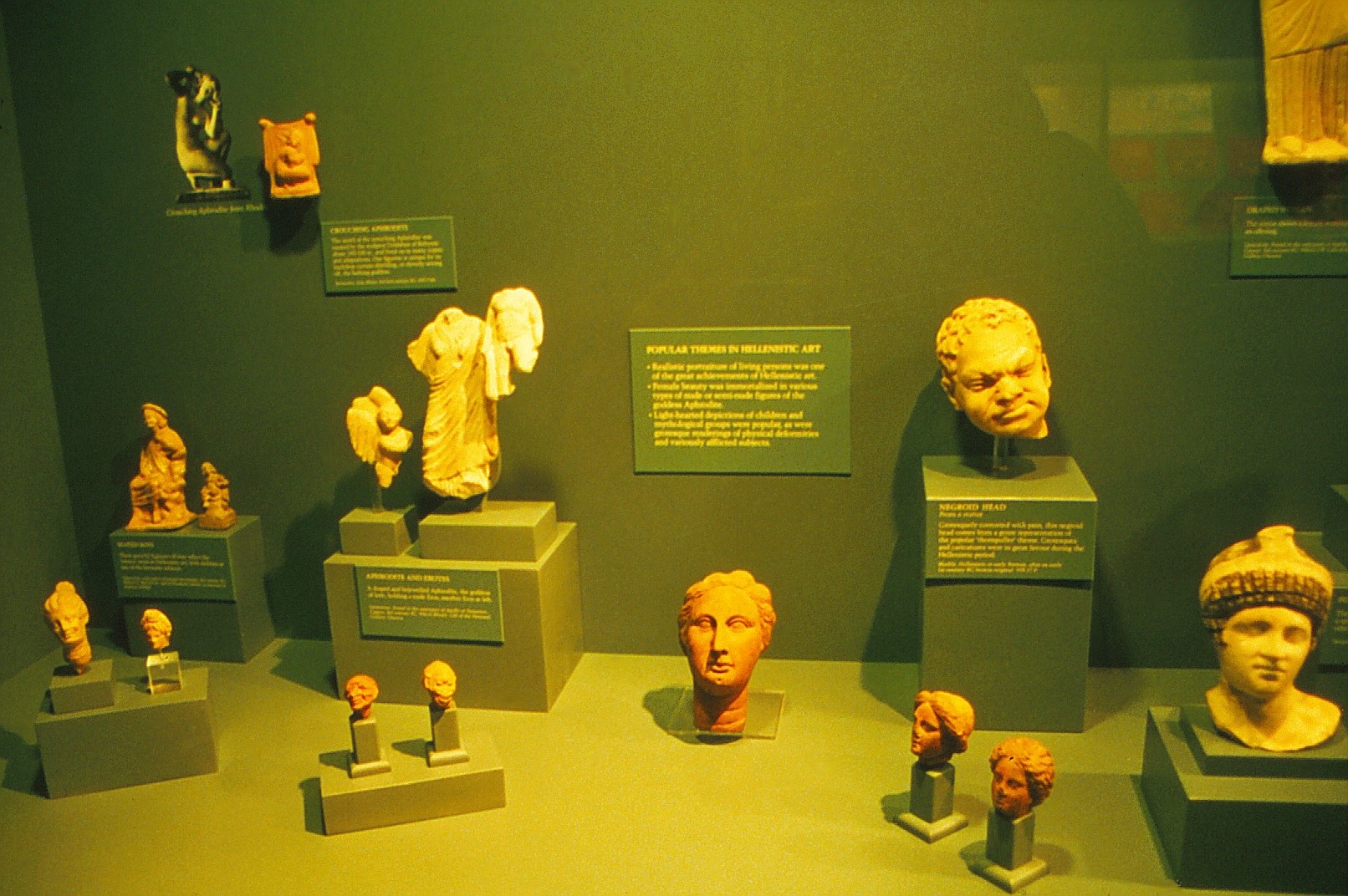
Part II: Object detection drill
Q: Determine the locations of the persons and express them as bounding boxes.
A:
[344,674,379,718]
[140,609,173,650]
[421,661,456,709]
[1200,527,1342,750]
[408,307,502,499]
[165,68,232,189]
[200,461,230,509]
[276,132,311,186]
[912,691,976,764]
[346,388,413,488]
[130,403,188,523]
[44,581,92,675]
[677,570,777,734]
[991,739,1054,819]
[935,297,1052,439]
[480,288,544,400]
